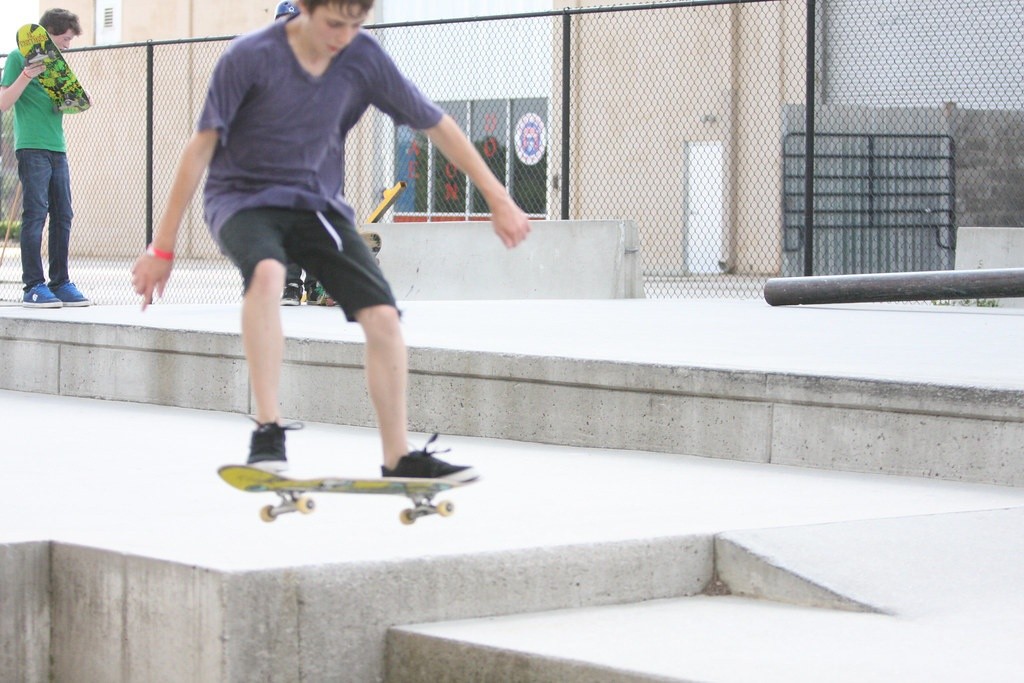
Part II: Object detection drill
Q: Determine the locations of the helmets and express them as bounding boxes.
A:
[276,0,300,15]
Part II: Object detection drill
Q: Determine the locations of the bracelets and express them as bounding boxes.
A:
[23,69,33,79]
[147,242,175,260]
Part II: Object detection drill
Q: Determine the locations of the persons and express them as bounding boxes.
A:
[277,2,336,305]
[0,8,91,309]
[125,0,534,483]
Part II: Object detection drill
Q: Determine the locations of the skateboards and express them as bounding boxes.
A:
[217,462,484,523]
[15,23,91,115]
[319,230,381,307]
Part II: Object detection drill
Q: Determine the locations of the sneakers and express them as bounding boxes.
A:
[280,278,303,306]
[246,415,303,472]
[381,433,479,483]
[23,283,63,307]
[47,282,89,306]
[306,284,337,305]
